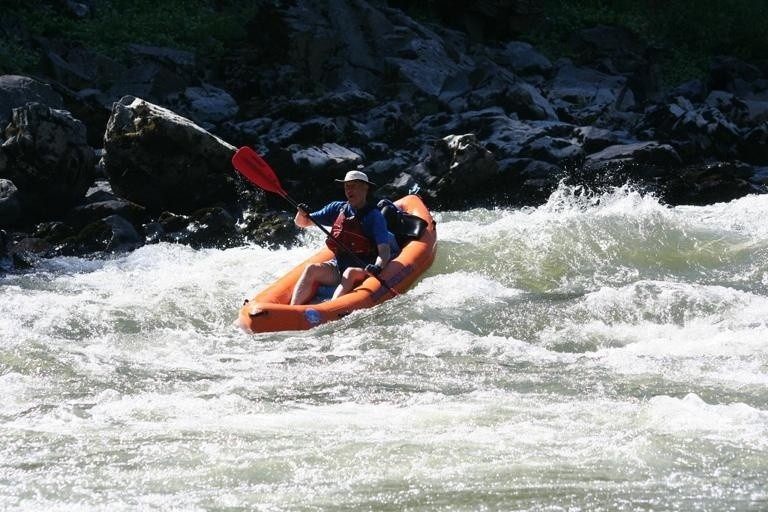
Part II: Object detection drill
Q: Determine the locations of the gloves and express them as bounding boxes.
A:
[364,263,383,277]
[296,202,311,218]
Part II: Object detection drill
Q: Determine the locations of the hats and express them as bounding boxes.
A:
[335,170,377,190]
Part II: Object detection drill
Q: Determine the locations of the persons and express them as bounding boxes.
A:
[289,169,402,305]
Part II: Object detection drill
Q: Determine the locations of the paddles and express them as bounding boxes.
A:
[232,146,402,297]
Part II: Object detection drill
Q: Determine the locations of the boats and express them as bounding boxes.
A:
[236,191,439,333]
